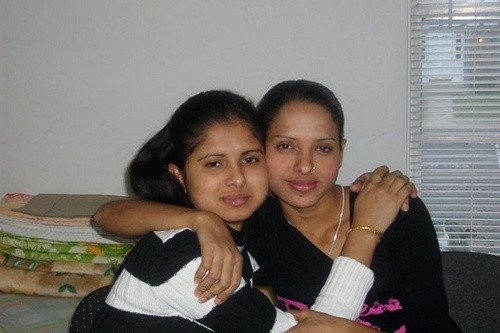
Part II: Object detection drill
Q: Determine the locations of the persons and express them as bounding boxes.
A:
[92,80,460,333]
[90,91,418,333]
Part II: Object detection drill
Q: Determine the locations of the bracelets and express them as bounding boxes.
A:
[345,225,383,239]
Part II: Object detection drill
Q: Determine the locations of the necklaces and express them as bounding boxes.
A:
[284,186,345,257]
